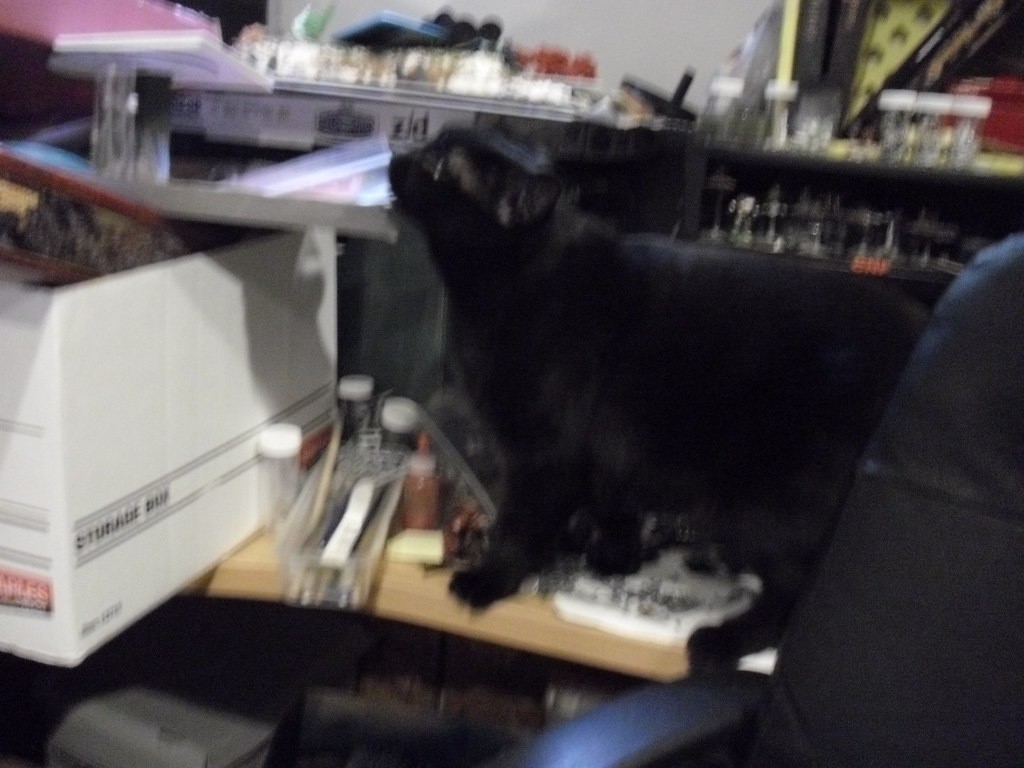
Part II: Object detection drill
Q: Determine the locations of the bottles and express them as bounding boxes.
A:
[254,423,303,538]
[404,430,440,529]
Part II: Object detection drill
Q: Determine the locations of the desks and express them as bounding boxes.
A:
[197,531,788,696]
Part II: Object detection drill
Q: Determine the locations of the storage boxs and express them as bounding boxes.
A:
[941,73,1024,153]
[166,1,779,155]
[0,175,344,668]
